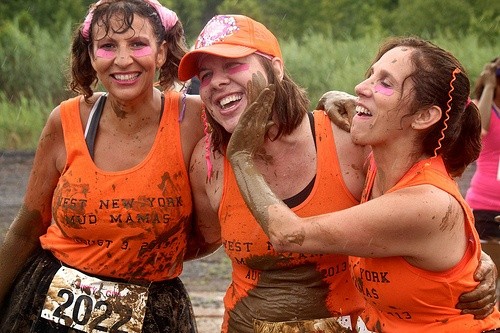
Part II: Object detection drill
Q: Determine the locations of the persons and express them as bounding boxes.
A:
[184,14,499,333]
[466,59,500,278]
[226,37,500,331]
[0,1,357,333]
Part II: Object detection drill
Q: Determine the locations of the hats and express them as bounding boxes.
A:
[178,14,283,82]
